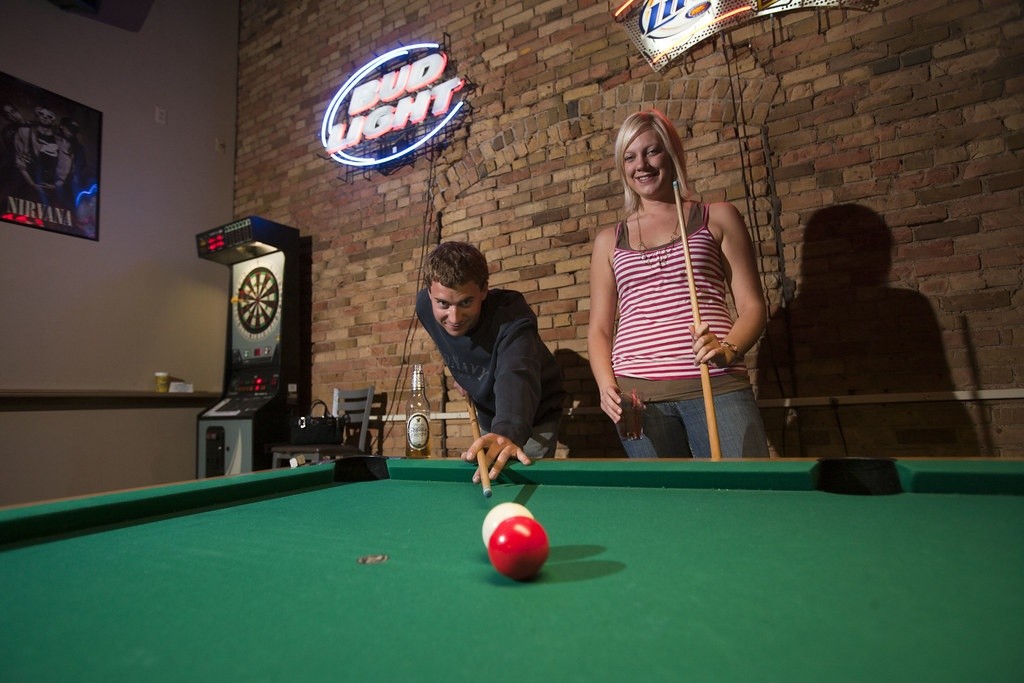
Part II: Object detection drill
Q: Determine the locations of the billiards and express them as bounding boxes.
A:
[481,502,536,550]
[488,516,551,581]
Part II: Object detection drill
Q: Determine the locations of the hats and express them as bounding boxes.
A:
[61,118,80,133]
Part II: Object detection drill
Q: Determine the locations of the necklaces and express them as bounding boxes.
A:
[636,208,679,271]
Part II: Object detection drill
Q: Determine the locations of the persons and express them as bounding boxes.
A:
[416,241,564,483]
[0,101,95,239]
[588,109,767,459]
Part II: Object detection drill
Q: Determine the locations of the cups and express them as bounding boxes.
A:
[614,391,644,441]
[155,372,169,393]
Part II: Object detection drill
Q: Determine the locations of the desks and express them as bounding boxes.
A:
[0,453,1024,683]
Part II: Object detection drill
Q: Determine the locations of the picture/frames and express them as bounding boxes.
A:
[0,71,104,241]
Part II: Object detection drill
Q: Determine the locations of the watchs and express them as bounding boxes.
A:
[721,342,738,368]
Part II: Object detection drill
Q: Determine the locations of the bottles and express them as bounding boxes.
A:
[405,363,432,459]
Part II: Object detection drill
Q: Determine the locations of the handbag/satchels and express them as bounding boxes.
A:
[290,399,344,444]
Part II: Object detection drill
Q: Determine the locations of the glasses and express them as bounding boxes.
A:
[38,110,55,120]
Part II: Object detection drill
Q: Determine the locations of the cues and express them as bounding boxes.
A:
[671,178,723,459]
[464,392,494,499]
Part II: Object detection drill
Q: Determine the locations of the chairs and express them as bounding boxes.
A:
[272,385,375,468]
